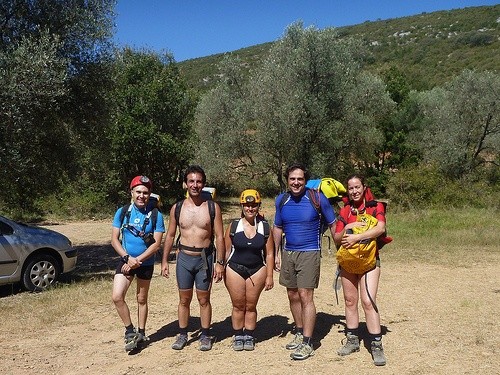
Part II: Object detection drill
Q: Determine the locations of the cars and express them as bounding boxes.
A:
[0,216,78,291]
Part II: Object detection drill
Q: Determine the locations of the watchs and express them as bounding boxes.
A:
[123,254,129,261]
[217,260,224,265]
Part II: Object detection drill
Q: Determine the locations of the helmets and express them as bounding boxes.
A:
[130,176,152,190]
[240,189,261,204]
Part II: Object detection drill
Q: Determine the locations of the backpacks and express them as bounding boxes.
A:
[279,189,341,233]
[175,187,216,228]
[120,193,161,229]
[338,201,387,248]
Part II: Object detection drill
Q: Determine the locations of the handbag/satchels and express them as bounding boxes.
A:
[336,213,378,274]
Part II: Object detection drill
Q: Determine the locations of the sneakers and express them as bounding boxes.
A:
[172,334,188,350]
[137,336,147,349]
[286,332,303,350]
[243,335,255,350]
[337,335,360,355]
[233,336,243,350]
[200,335,212,350]
[124,331,141,349]
[371,341,386,366]
[290,342,314,359]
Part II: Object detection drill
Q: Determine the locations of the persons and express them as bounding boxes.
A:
[161,164,225,351]
[272,164,338,360]
[333,174,387,366]
[223,189,275,351]
[111,175,165,355]
